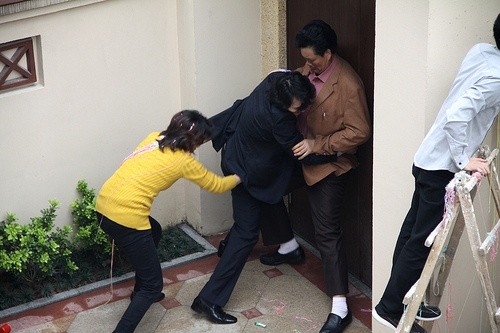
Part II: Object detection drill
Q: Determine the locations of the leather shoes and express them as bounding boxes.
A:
[319,305,352,333]
[190,296,238,324]
[260,244,305,265]
[217,240,226,257]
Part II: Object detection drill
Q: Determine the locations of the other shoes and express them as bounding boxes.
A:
[404,302,442,321]
[130,292,165,302]
[375,306,426,333]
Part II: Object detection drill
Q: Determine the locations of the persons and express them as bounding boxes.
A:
[373,13,500,333]
[94,109,243,333]
[189,18,372,333]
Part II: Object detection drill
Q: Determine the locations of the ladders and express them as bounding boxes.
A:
[392,145,500,333]
